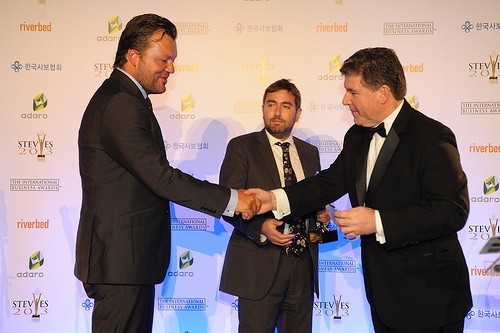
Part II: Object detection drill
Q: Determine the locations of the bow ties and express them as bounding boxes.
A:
[361,122,387,139]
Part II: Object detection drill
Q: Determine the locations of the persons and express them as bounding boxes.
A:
[218,79,335,333]
[73,11,262,333]
[240,46,474,333]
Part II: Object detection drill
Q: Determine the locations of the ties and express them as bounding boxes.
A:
[146,96,153,111]
[276,141,307,258]
